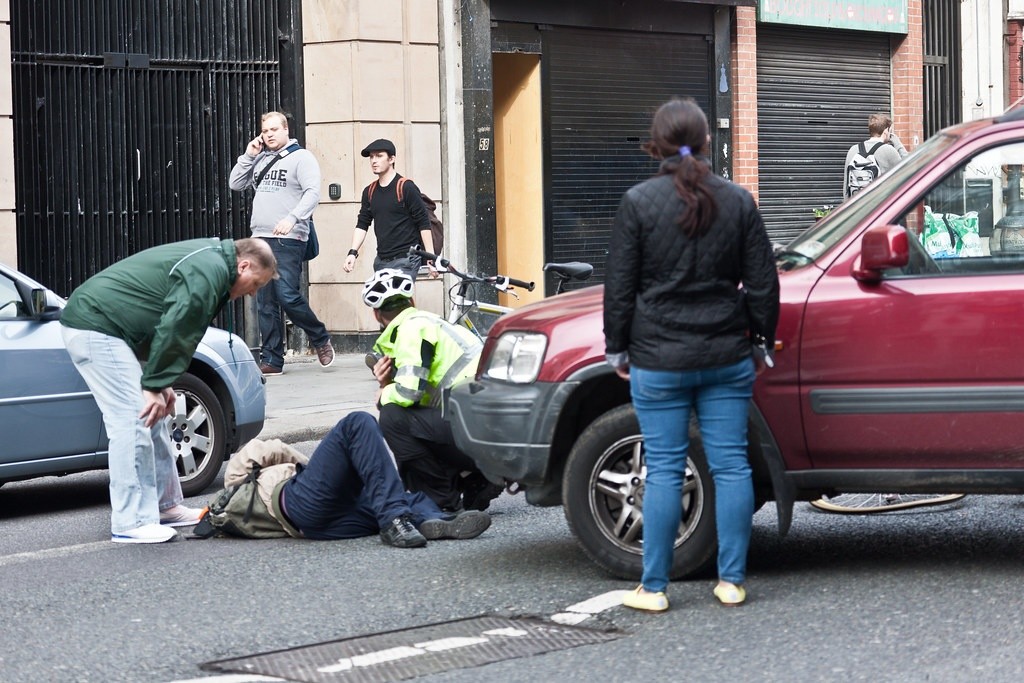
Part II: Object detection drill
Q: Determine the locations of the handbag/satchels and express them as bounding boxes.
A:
[462,477,505,512]
[305,216,319,260]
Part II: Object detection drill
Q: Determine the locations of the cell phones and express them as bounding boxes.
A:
[261,138,267,146]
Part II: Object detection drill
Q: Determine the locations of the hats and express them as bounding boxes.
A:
[361,138,396,158]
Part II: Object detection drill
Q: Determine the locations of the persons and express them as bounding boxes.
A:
[227,112,334,376]
[362,268,507,512]
[223,410,492,547]
[603,98,780,613]
[58,238,277,545]
[340,139,439,335]
[843,114,907,229]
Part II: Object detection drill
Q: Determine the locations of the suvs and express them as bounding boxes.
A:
[441,102,1023,584]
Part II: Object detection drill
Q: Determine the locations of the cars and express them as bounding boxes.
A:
[0,263,267,497]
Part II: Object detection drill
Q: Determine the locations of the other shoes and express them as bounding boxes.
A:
[712,580,745,606]
[623,584,669,613]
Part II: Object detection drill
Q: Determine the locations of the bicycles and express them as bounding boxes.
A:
[409,244,593,347]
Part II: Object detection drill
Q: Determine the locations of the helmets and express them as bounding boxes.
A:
[361,267,413,308]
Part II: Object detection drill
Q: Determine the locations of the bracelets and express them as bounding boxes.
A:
[348,249,359,258]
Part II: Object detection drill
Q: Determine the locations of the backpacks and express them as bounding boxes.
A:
[846,141,885,198]
[368,178,443,266]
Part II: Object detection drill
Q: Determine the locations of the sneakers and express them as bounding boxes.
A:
[258,363,283,375]
[420,510,492,539]
[111,521,178,543]
[379,516,428,548]
[316,339,336,368]
[160,504,204,526]
